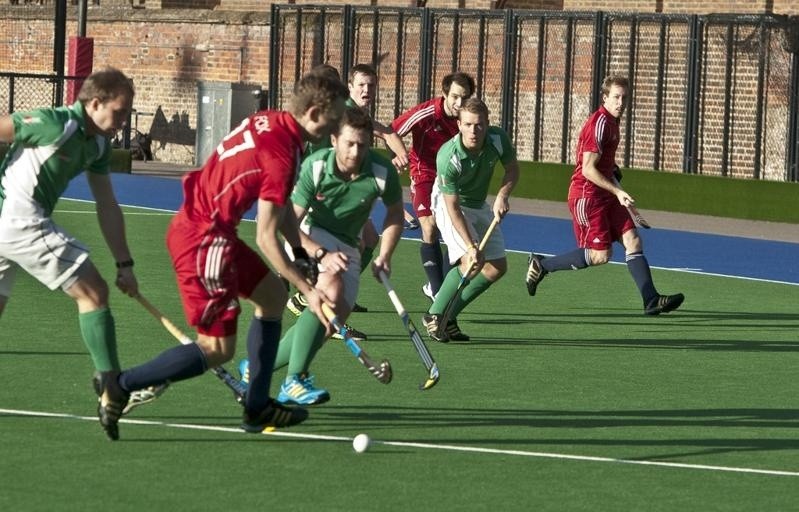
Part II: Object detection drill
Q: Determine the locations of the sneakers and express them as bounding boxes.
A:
[238,360,331,434]
[644,293,684,315]
[352,302,368,311]
[332,323,366,341]
[526,253,548,295]
[421,282,469,343]
[92,371,130,440]
[121,379,169,414]
[286,292,308,317]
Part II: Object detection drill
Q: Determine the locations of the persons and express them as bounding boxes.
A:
[235,108,404,406]
[526,72,684,318]
[0,68,169,416]
[339,64,409,311]
[92,67,350,441]
[385,73,479,302]
[421,98,520,341]
[275,66,367,342]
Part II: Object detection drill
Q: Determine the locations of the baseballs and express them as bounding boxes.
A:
[353,433,371,452]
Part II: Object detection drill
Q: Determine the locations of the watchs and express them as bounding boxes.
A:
[315,247,327,262]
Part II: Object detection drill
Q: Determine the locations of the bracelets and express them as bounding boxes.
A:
[291,247,310,261]
[116,258,134,269]
